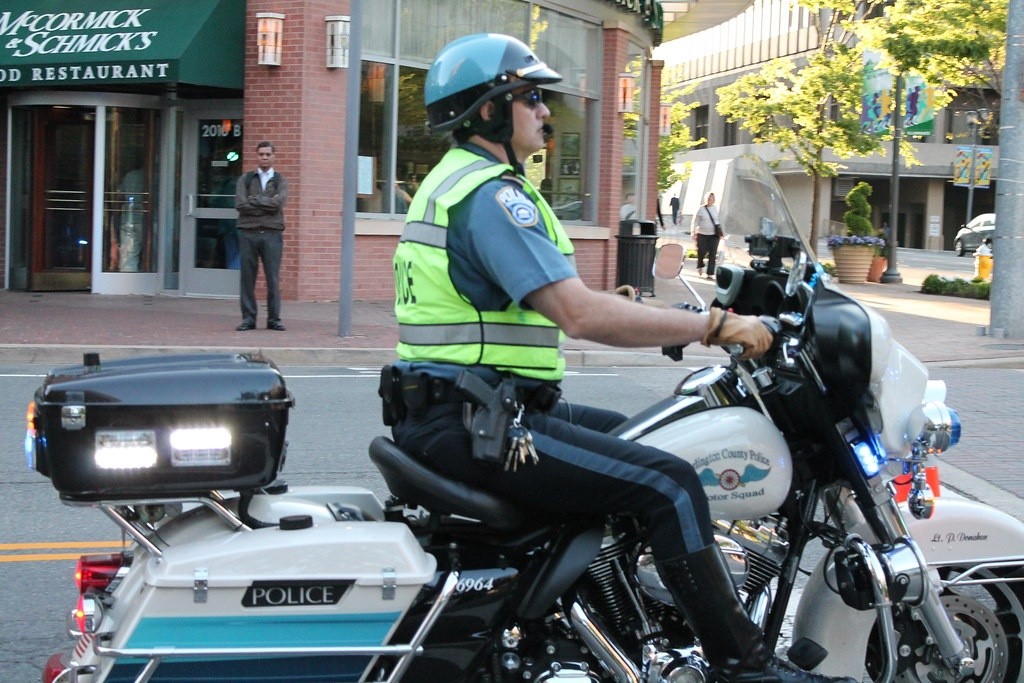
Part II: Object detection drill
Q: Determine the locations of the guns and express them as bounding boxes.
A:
[455,368,518,468]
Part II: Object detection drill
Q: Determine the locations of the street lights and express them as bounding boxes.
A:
[880,61,907,282]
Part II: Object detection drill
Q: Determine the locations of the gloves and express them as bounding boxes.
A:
[612,284,673,309]
[703,306,773,362]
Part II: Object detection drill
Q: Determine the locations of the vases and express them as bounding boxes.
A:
[833,244,877,285]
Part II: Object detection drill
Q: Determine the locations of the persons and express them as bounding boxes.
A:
[670,193,680,224]
[219,167,242,270]
[691,192,720,279]
[235,142,288,332]
[621,192,636,221]
[973,237,993,283]
[656,199,665,231]
[114,169,145,273]
[883,222,891,236]
[393,32,857,683]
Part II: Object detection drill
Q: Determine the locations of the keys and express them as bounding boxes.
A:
[503,420,539,472]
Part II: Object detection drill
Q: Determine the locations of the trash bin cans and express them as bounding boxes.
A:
[614,219,660,297]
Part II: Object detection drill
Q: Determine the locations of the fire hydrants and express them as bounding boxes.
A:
[972,242,994,283]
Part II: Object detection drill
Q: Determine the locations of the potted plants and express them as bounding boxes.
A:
[842,182,888,282]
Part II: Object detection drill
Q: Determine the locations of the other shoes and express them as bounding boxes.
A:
[699,267,712,281]
[267,320,286,331]
[236,322,256,331]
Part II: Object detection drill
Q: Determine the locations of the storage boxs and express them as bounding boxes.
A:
[24,351,296,509]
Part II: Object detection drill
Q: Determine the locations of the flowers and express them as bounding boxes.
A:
[827,235,886,250]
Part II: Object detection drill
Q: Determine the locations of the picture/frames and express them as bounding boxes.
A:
[557,178,581,203]
[560,132,581,157]
[559,159,581,176]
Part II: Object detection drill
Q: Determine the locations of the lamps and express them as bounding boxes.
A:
[256,12,286,67]
[658,103,673,137]
[617,73,638,113]
[324,15,351,70]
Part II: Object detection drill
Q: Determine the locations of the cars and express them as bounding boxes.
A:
[953,213,997,256]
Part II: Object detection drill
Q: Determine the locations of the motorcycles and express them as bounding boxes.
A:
[23,152,1024,682]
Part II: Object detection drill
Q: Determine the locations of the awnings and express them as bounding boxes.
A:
[0,0,245,90]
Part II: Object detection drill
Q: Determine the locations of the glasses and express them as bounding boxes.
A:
[513,88,544,106]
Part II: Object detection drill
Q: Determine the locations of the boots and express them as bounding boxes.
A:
[656,542,859,683]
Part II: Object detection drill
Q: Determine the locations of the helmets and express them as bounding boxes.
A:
[425,35,563,133]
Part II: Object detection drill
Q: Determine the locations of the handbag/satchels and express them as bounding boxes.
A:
[716,224,724,238]
